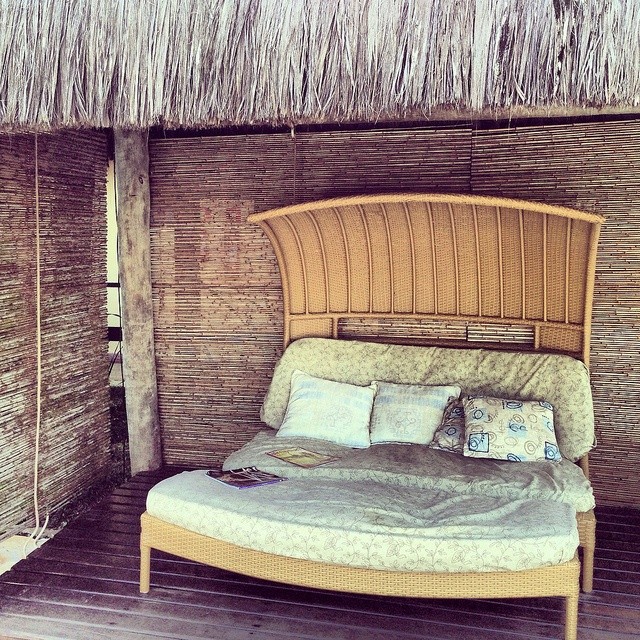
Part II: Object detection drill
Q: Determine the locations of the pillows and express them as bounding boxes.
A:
[370,379,460,445]
[274,370,375,450]
[429,396,464,453]
[461,395,562,462]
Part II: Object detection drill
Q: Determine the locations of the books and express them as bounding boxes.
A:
[205,466,289,490]
[265,445,341,470]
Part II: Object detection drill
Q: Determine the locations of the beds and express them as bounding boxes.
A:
[139,191,606,640]
